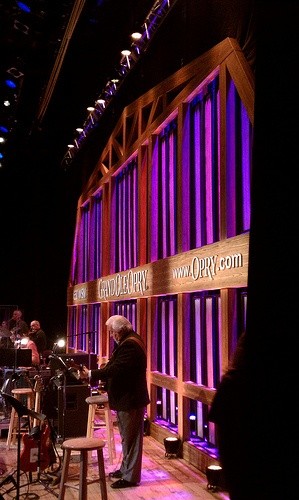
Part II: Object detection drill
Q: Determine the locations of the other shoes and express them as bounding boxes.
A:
[110,478,141,488]
[109,470,122,478]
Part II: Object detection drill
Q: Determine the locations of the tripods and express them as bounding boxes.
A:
[6,421,59,498]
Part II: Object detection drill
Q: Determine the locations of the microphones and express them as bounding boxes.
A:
[8,317,14,322]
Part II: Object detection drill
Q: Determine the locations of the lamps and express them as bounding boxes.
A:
[67,19,149,148]
[206,464,224,493]
[143,417,148,433]
[54,330,99,384]
[0,0,45,169]
[164,436,182,459]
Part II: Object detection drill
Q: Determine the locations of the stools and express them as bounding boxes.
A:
[85,395,117,465]
[6,388,35,451]
[58,437,108,500]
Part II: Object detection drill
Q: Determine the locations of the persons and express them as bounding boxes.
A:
[78,315,150,488]
[0,310,46,365]
[207,332,246,500]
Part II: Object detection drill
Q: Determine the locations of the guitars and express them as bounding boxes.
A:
[64,356,108,394]
[19,375,49,476]
